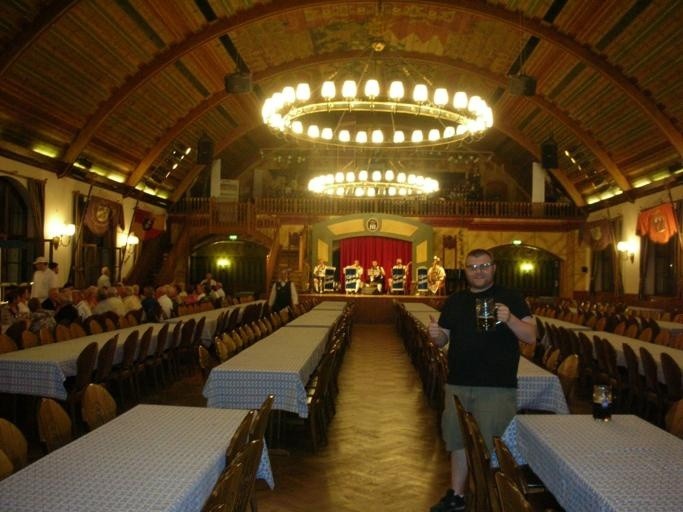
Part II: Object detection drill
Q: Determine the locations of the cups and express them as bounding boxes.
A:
[593,385,613,420]
[474,297,502,332]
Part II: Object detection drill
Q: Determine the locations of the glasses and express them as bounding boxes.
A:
[465,262,492,270]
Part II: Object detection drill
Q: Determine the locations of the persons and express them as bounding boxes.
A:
[0,256,227,335]
[312,259,326,293]
[426,255,446,293]
[266,266,298,315]
[426,247,539,512]
[344,259,365,289]
[387,257,409,295]
[370,261,386,293]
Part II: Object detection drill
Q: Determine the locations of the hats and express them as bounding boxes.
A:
[33,257,48,264]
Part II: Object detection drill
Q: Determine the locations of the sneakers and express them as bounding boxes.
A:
[430,488,466,512]
